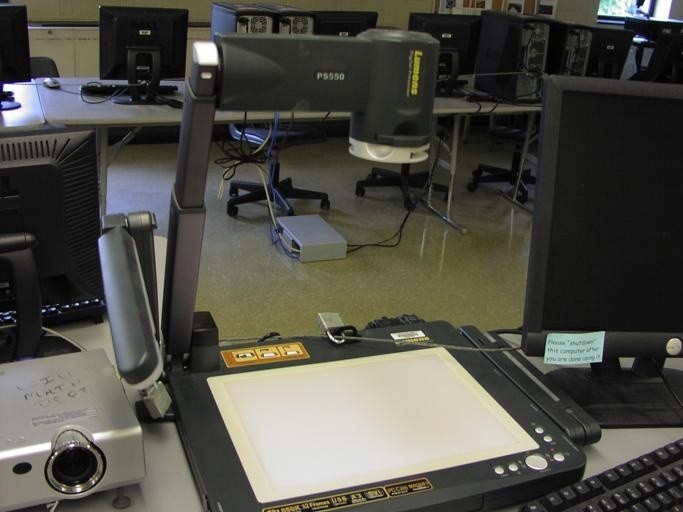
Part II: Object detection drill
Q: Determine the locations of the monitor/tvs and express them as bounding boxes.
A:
[1,4,32,103]
[520,72,683,430]
[0,128,104,352]
[409,12,482,100]
[309,11,380,36]
[99,5,188,112]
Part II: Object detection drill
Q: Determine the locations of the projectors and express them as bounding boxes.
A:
[0,348,146,512]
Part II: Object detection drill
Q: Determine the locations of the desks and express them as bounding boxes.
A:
[0,82,45,134]
[33,77,542,234]
[0,236,205,512]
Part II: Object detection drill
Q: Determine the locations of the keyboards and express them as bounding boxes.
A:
[499,439,683,511]
[79,79,182,96]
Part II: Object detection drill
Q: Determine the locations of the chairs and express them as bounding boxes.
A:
[355,163,454,211]
[226,123,330,217]
[467,113,576,203]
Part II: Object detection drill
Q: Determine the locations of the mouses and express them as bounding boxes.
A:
[43,78,60,90]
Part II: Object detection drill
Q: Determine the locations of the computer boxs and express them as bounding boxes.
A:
[211,1,280,42]
[258,3,314,36]
[476,11,683,107]
